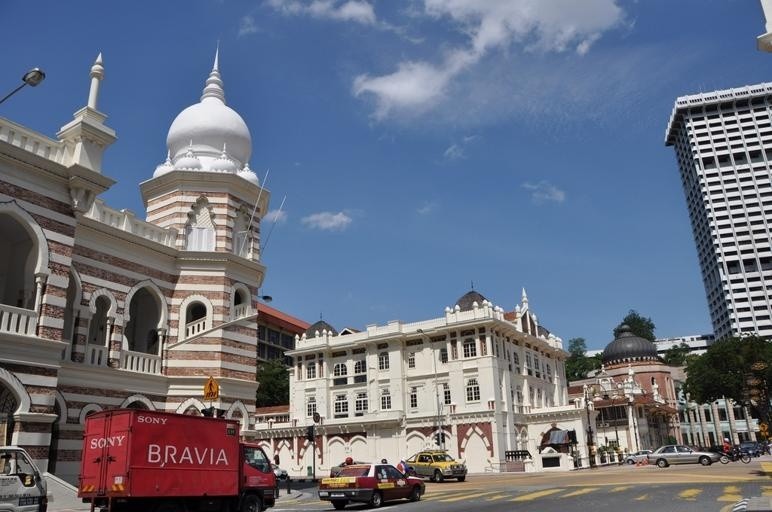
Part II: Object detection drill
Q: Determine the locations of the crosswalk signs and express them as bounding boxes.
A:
[203,377,219,400]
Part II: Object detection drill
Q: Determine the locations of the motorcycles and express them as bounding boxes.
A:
[719,447,752,464]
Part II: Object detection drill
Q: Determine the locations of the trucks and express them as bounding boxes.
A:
[0,444,48,512]
[78,409,280,512]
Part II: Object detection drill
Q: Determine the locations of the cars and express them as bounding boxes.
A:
[403,450,468,483]
[648,444,719,469]
[624,449,654,466]
[318,464,426,510]
[271,463,289,484]
[739,441,762,457]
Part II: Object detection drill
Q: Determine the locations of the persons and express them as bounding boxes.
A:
[723,438,735,462]
[346,457,353,465]
[382,458,387,464]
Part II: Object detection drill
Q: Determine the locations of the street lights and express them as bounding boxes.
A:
[0,64,45,107]
[612,395,634,462]
[672,413,679,443]
[416,328,443,453]
[585,382,610,466]
[258,295,273,304]
[645,404,661,452]
